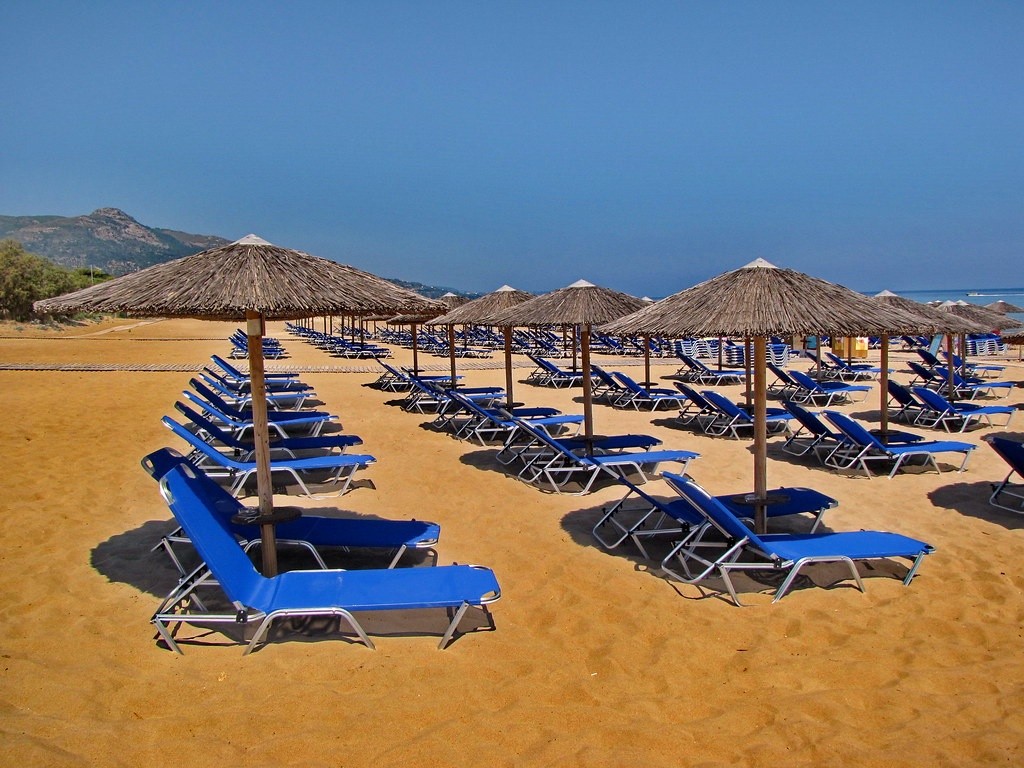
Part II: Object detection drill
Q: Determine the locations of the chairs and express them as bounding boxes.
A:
[935,368,1017,401]
[912,387,1018,434]
[139,320,980,657]
[976,333,1008,356]
[940,352,1007,379]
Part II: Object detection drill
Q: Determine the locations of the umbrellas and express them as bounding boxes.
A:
[32,232,1023,576]
